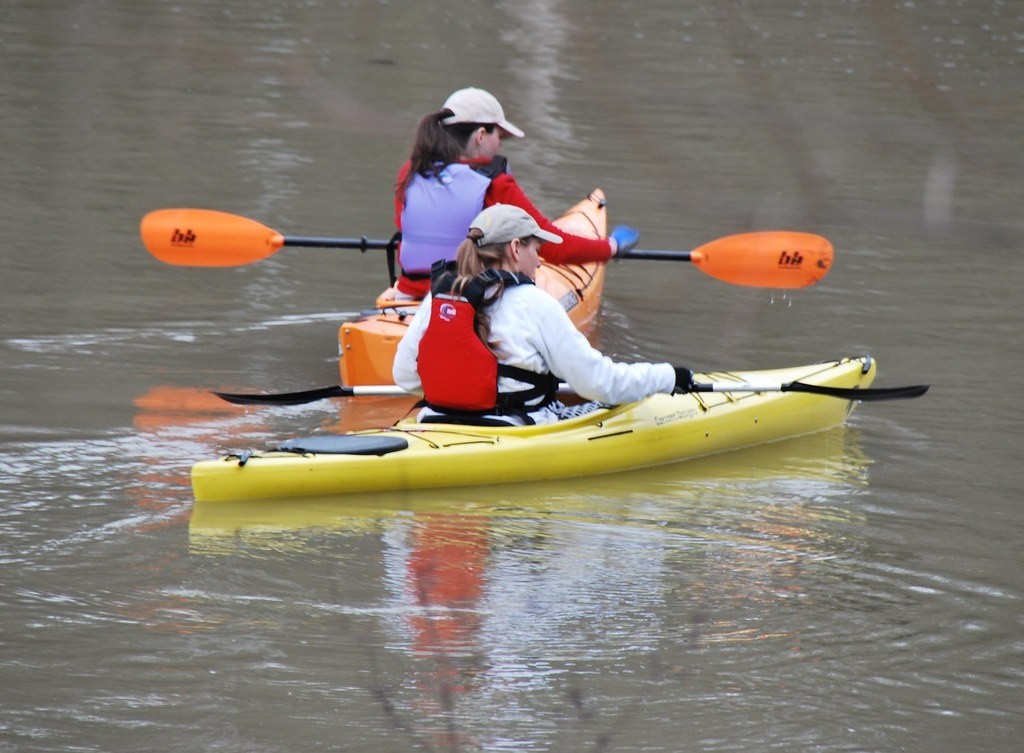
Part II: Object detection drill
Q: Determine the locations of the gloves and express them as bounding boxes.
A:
[611,225,640,259]
[671,367,694,396]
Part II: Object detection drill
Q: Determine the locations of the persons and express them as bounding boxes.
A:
[393,86,695,427]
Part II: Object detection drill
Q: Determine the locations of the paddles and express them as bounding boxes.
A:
[208,378,931,402]
[137,206,835,291]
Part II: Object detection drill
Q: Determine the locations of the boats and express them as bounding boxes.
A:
[333,187,610,391]
[187,427,871,542]
[190,355,878,512]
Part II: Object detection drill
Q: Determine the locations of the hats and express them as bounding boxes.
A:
[468,202,563,246]
[439,88,524,139]
